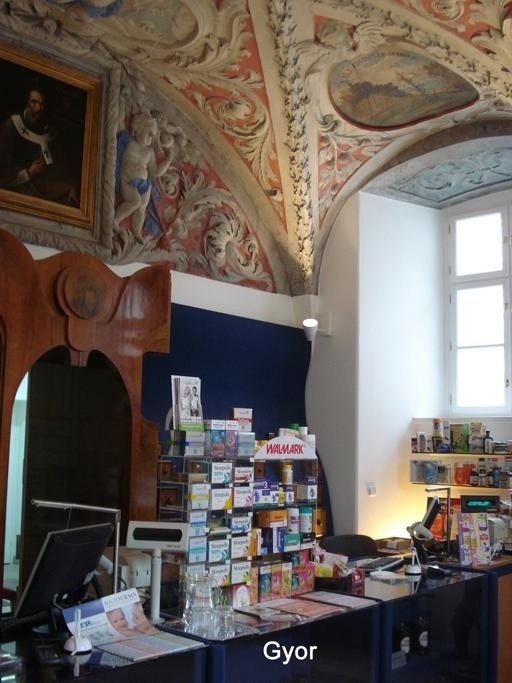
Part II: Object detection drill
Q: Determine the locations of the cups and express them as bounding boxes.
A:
[215,605,234,631]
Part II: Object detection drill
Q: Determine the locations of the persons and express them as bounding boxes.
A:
[191,386,201,418]
[6,88,78,207]
[178,385,192,419]
[131,603,161,634]
[104,607,146,638]
[114,111,176,246]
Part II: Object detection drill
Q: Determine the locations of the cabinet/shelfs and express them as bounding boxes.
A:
[407,412,512,494]
[157,451,330,614]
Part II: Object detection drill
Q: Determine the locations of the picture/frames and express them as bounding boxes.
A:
[0,37,103,232]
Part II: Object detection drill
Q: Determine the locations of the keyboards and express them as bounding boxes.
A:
[360,555,402,577]
[0,610,47,639]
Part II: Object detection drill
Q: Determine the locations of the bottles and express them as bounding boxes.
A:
[482,430,494,455]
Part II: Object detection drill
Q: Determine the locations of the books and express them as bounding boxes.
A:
[233,604,280,619]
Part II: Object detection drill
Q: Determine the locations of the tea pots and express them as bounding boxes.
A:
[182,573,220,630]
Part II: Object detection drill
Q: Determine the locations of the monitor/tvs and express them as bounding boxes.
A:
[405,495,440,575]
[16,523,114,637]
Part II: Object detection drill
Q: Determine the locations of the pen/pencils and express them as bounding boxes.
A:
[411,549,414,565]
[77,609,81,637]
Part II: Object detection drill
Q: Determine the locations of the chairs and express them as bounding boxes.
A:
[317,532,378,560]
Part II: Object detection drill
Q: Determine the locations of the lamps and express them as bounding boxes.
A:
[301,316,326,343]
